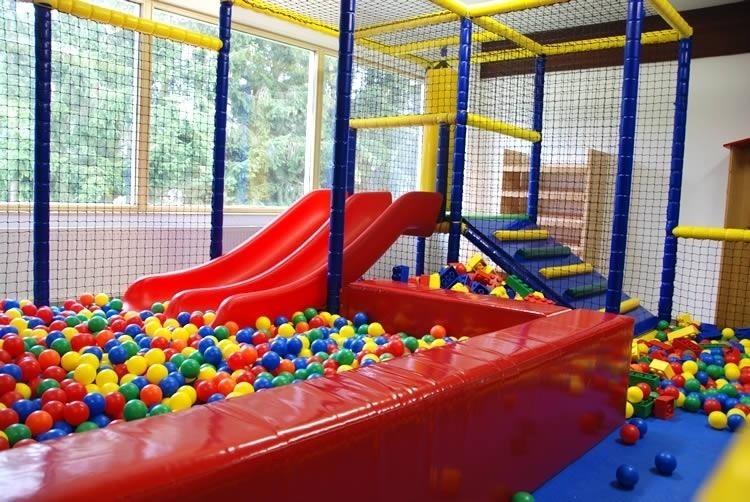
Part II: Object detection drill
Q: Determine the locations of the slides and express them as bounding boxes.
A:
[122,189,442,330]
